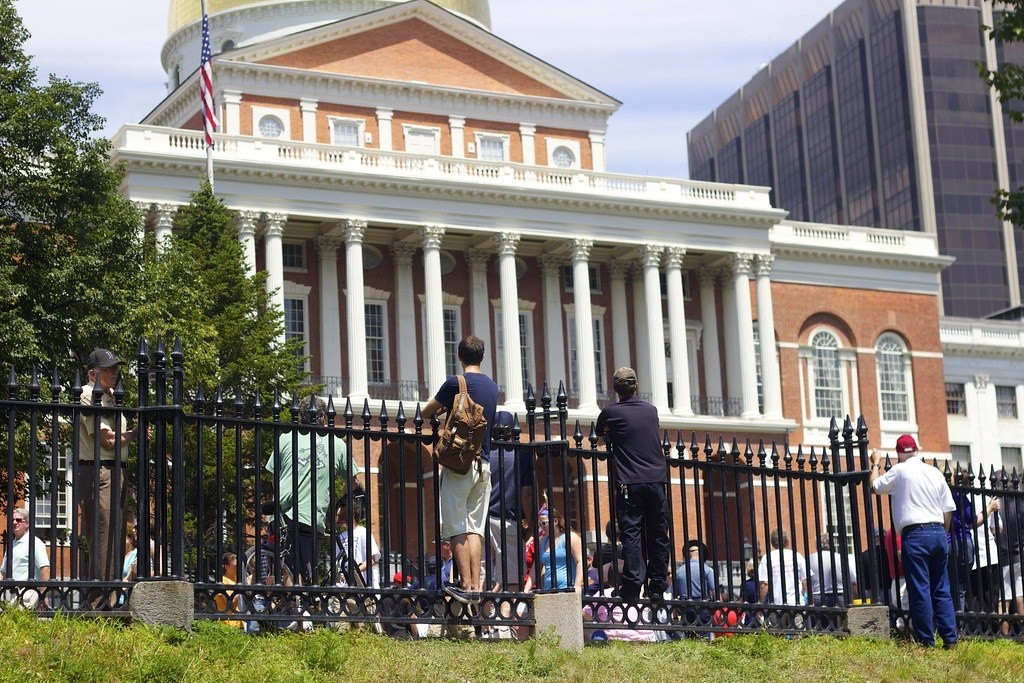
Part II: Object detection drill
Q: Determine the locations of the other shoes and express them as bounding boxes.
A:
[611,584,641,602]
[471,591,483,604]
[442,580,473,606]
[642,588,665,601]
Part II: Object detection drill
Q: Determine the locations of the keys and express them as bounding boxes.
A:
[621,484,628,501]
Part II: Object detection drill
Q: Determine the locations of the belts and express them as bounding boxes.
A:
[80,460,131,468]
[900,522,943,529]
[489,511,517,520]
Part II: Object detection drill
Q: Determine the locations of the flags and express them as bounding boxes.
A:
[198,0,218,146]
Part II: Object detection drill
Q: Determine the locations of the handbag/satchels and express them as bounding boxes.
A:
[429,375,488,475]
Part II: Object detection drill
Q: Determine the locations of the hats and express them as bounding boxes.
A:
[495,411,517,428]
[87,347,127,369]
[393,573,412,584]
[612,367,644,388]
[895,434,918,453]
[432,533,452,544]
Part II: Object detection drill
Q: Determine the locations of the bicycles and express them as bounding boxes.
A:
[235,485,385,635]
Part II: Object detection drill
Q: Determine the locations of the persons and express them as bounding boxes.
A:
[265,395,360,589]
[595,367,672,604]
[870,434,958,651]
[0,508,50,611]
[121,483,455,637]
[478,411,534,638]
[421,335,498,604]
[78,349,152,611]
[938,466,1024,637]
[525,505,913,642]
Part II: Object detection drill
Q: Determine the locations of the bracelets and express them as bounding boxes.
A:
[871,464,881,471]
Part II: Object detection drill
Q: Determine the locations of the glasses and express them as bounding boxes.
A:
[540,521,549,526]
[12,518,27,523]
